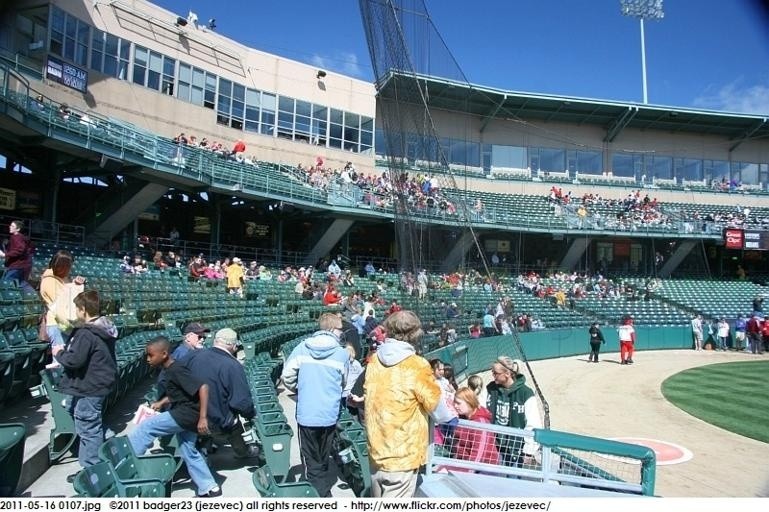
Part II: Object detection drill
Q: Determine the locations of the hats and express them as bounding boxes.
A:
[184,322,210,333]
[214,328,242,346]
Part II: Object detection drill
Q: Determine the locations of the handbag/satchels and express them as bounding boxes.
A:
[38,314,51,343]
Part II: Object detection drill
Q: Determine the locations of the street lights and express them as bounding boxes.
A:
[618,0,666,107]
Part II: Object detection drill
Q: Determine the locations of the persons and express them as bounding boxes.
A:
[0,92,769,498]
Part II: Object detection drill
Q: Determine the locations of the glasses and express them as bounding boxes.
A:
[196,335,207,340]
